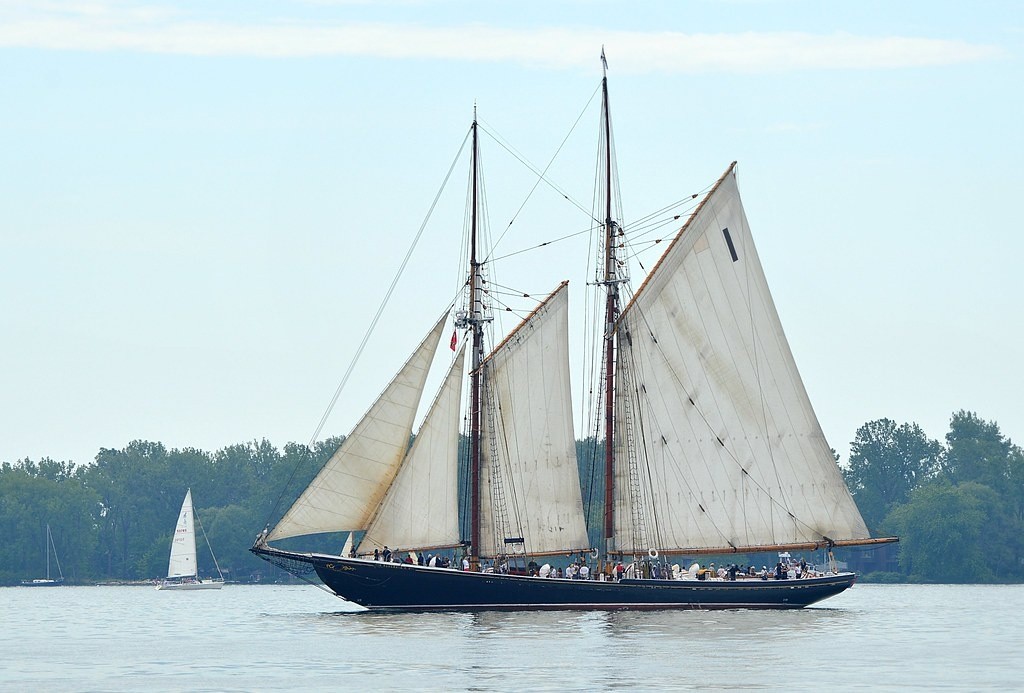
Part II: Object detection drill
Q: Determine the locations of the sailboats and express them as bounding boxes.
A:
[157,487,225,591]
[19,524,64,586]
[247,40,902,613]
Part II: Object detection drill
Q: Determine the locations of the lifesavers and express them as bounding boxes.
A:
[648,547,659,559]
[590,548,598,559]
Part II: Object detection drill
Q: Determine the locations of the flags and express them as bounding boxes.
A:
[450,330,457,351]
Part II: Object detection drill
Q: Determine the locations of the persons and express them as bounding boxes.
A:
[350,544,356,558]
[382,545,391,562]
[374,548,378,560]
[405,554,412,564]
[426,553,826,584]
[418,552,423,566]
